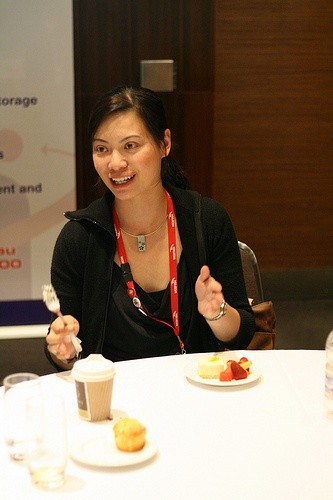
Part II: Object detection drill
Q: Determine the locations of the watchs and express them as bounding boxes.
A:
[202,297,227,321]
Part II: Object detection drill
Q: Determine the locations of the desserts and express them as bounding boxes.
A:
[197,355,224,379]
[113,418,146,451]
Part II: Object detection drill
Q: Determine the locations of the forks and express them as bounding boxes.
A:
[42,283,85,352]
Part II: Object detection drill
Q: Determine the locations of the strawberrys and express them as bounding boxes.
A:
[218,357,250,380]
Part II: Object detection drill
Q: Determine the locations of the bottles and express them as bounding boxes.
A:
[323,324,333,417]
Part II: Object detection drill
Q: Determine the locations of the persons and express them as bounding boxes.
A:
[44,87,259,371]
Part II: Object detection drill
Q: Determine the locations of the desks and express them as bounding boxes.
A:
[0,349,333,500]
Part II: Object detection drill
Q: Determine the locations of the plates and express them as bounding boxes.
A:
[66,423,159,468]
[185,360,262,386]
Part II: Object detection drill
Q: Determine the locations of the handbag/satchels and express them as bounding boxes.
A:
[225,300,277,351]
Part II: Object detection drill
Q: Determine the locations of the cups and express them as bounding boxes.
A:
[71,352,117,421]
[3,369,64,487]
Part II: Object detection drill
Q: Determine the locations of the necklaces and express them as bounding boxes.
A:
[118,211,169,253]
